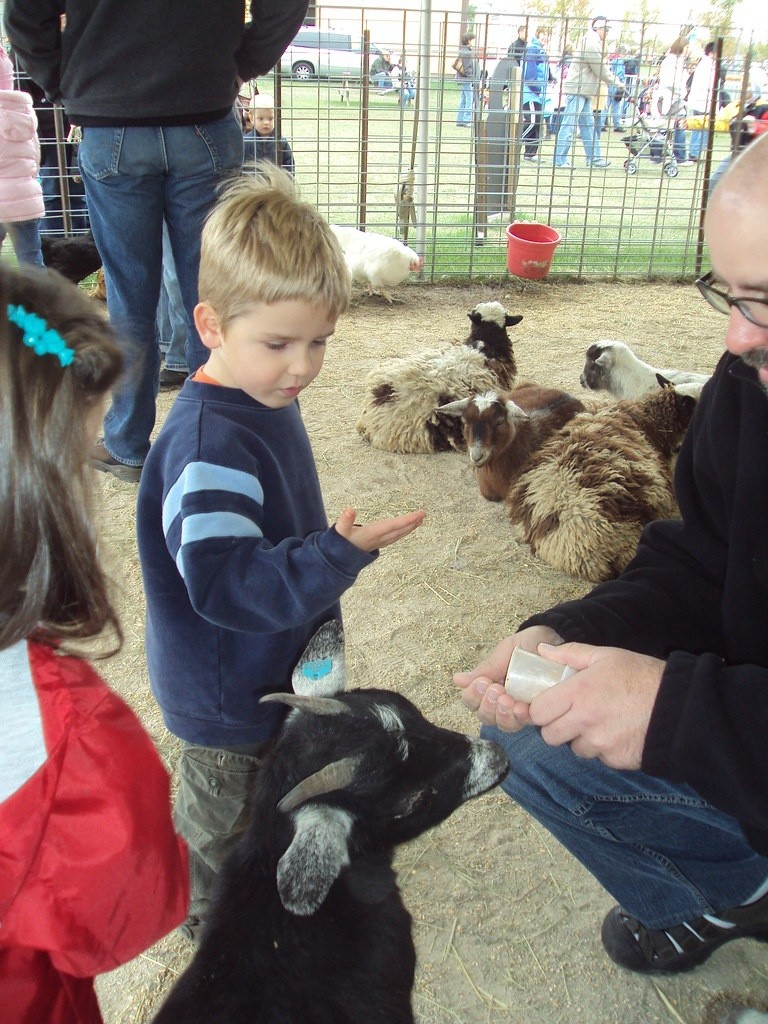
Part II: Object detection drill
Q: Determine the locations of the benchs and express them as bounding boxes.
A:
[338,86,398,97]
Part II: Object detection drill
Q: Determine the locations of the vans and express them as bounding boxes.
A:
[269,27,400,82]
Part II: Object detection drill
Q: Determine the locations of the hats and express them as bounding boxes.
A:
[593,20,612,28]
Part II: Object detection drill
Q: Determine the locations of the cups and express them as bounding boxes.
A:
[503,646,580,706]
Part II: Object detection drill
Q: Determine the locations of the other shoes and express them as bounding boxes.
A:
[555,162,576,167]
[158,369,188,391]
[88,437,143,483]
[525,156,546,162]
[677,161,693,166]
[587,159,611,167]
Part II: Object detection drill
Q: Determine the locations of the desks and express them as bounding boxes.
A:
[324,74,418,106]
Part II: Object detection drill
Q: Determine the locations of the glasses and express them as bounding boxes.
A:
[694,269,768,328]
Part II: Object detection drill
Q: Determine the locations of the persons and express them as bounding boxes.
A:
[455,128,768,976]
[137,159,429,945]
[453,16,768,201]
[0,0,308,483]
[369,49,416,108]
[0,261,190,1024]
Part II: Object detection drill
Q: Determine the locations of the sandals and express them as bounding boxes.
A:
[601,887,768,976]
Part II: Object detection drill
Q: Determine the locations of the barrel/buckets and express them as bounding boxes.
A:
[506,222,562,278]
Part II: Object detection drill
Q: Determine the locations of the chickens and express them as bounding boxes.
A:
[325,227,425,304]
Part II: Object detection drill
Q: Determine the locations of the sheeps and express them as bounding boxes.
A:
[150,686,510,1024]
[354,300,712,587]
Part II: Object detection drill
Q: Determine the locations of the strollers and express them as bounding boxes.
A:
[620,84,690,178]
